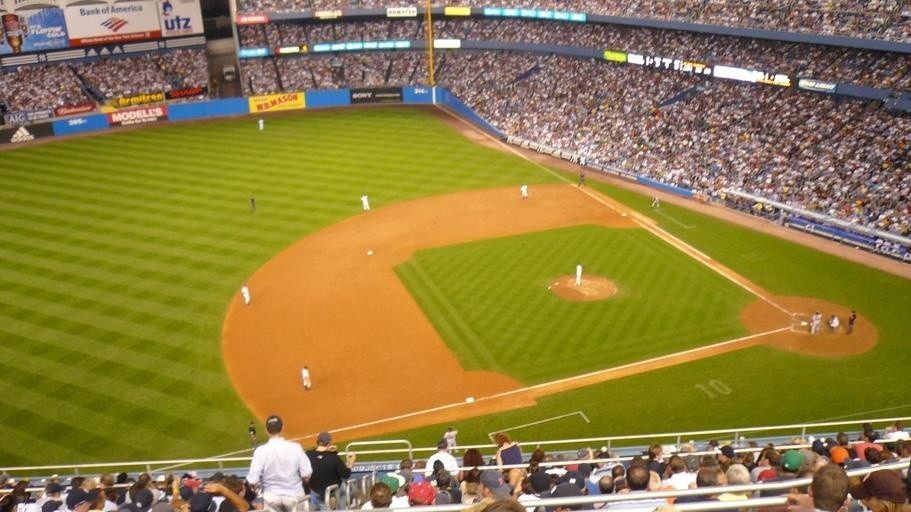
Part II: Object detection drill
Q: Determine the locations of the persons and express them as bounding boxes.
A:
[0,47,212,125]
[846,311,857,334]
[0,416,911,510]
[249,195,256,212]
[242,285,250,305]
[258,117,265,130]
[302,366,311,390]
[235,0,446,93]
[361,194,371,214]
[829,315,839,328]
[425,1,911,237]
[811,311,822,334]
[576,262,581,285]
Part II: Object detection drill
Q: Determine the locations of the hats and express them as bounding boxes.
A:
[831,446,850,465]
[40,477,214,512]
[374,467,584,508]
[849,467,906,504]
[317,431,331,444]
[266,415,283,434]
[781,449,804,473]
